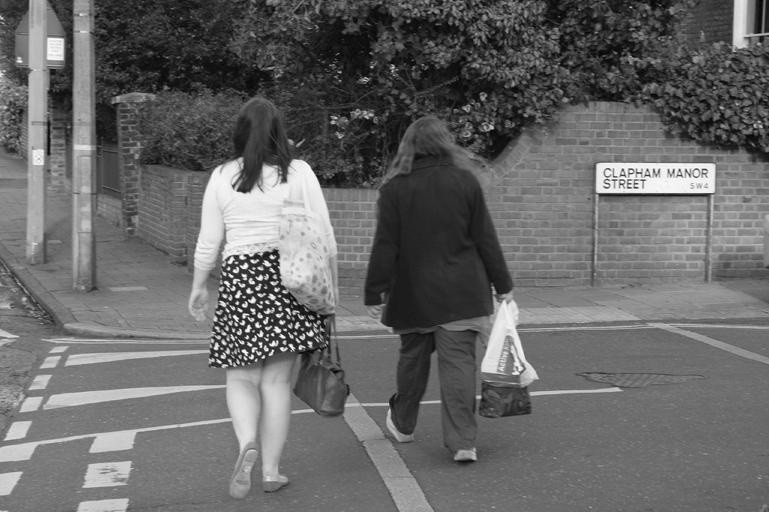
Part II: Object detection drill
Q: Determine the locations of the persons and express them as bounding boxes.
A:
[364,115,514,462]
[187,96,340,499]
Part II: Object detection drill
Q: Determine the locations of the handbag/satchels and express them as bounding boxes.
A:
[478,297,539,418]
[278,159,336,316]
[292,314,350,418]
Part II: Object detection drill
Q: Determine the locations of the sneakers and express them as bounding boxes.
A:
[385,406,414,443]
[454,447,478,463]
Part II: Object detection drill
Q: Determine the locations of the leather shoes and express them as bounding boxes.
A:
[262,472,290,493]
[229,441,258,500]
